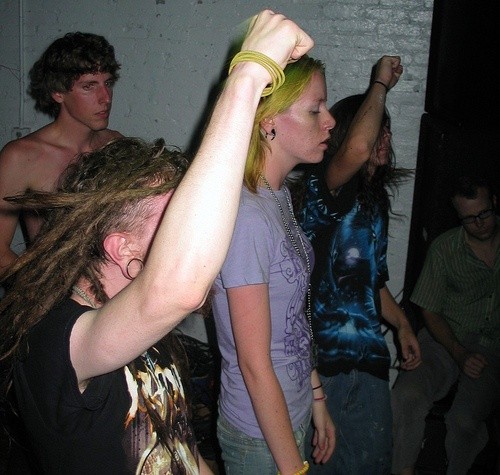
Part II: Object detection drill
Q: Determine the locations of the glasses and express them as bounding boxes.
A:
[460,198,495,225]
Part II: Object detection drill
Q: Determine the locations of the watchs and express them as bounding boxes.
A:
[310,383,324,390]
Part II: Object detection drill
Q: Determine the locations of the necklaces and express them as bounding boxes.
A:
[259,170,315,344]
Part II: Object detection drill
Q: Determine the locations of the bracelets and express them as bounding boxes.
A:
[314,394,328,401]
[371,78,388,93]
[225,48,287,97]
[276,460,309,475]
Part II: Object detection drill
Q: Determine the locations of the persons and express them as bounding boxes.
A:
[2,9,318,475]
[206,45,339,475]
[298,53,422,475]
[388,167,500,475]
[1,32,133,280]
